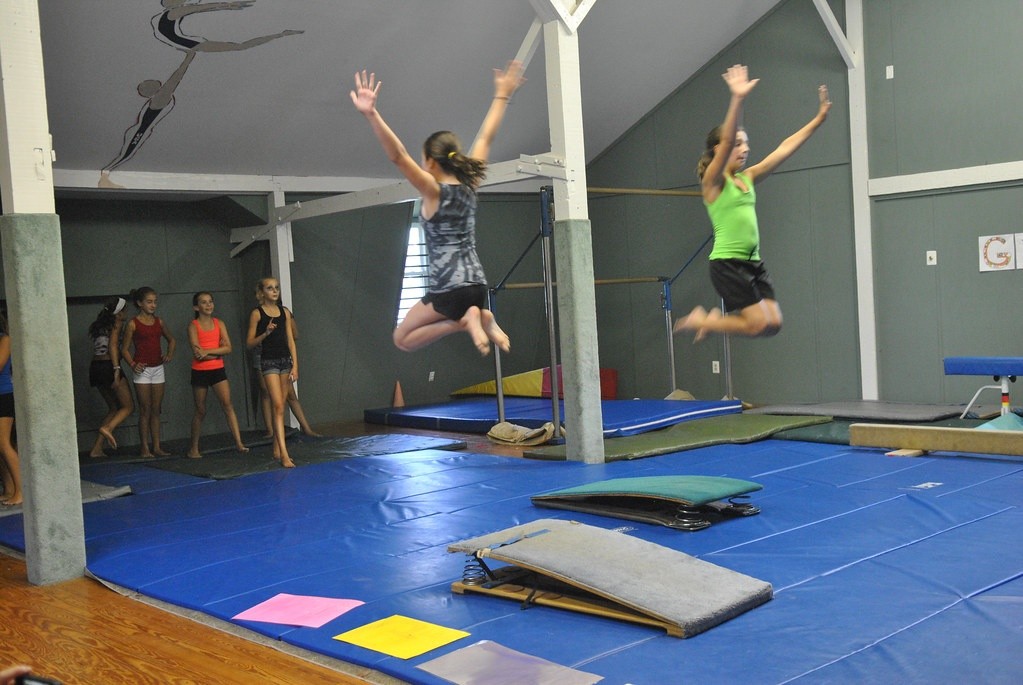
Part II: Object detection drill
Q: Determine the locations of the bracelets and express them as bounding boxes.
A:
[113,366,121,369]
[492,94,514,104]
[129,360,134,365]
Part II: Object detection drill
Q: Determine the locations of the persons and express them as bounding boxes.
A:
[349,58,528,356]
[89,296,136,458]
[187,291,250,458]
[672,64,832,345]
[121,287,176,457]
[246,277,299,467]
[252,280,322,439]
[0,312,23,504]
[0,662,33,685]
